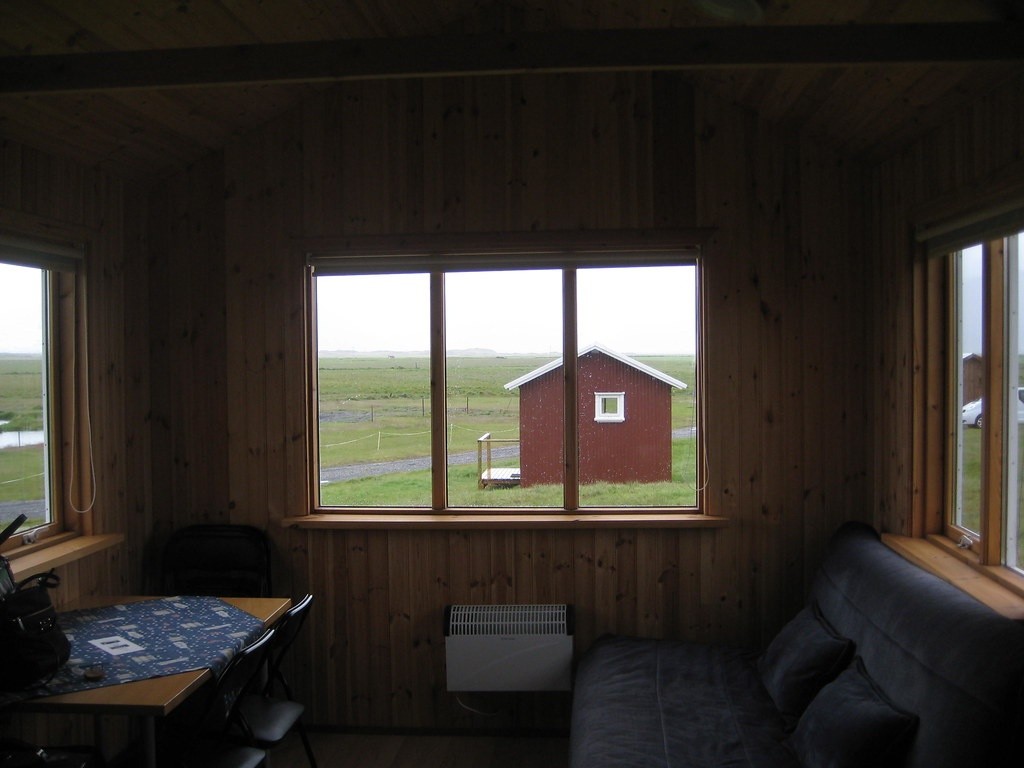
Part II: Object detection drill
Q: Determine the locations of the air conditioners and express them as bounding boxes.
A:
[444,601,573,694]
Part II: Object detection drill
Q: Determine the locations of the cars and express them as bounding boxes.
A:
[961,386,1024,430]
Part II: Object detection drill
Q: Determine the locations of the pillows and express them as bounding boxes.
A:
[755,600,853,732]
[780,655,919,768]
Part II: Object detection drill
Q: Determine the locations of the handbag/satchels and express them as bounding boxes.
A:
[0,567,72,693]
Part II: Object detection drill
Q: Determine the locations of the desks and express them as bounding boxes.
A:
[27,592,291,768]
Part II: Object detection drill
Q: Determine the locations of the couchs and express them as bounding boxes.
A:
[571,517,1024,768]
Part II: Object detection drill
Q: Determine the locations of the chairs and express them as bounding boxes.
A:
[1,527,319,768]
